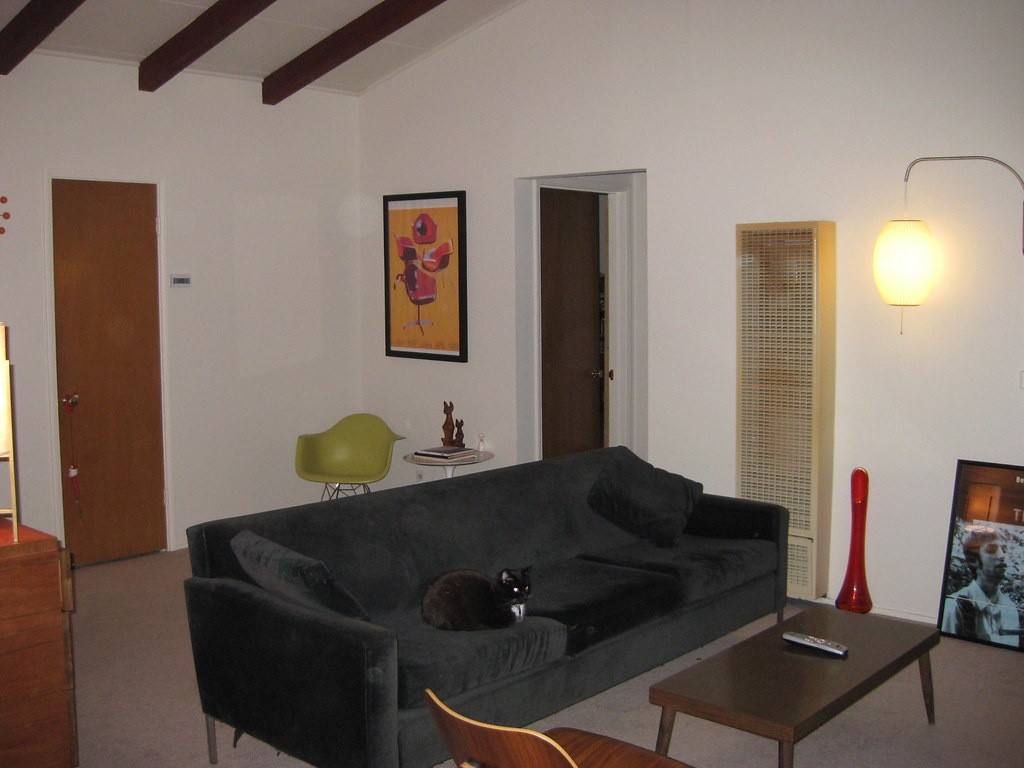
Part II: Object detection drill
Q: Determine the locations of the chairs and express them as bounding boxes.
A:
[295,413,407,503]
[426,687,694,768]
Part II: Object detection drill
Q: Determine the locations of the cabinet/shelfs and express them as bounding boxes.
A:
[0,514,79,768]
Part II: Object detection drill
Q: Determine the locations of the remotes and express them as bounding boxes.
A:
[782,632,850,657]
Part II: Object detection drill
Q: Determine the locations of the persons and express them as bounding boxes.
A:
[942,528,1019,648]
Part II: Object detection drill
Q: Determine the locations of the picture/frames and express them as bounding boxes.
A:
[383,190,467,363]
[936,459,1024,652]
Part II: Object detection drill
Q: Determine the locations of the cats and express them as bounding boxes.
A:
[422,565,533,633]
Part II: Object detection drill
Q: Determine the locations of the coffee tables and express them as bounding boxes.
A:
[649,603,941,768]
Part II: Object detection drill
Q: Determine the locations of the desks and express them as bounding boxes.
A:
[402,448,495,478]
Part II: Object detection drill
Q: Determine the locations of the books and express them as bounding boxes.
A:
[414,446,477,462]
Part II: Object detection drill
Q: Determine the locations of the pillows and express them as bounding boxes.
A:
[229,529,371,623]
[585,447,703,549]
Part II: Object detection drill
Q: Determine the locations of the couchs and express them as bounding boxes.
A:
[184,446,789,768]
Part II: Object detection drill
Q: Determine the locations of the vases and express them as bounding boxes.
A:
[835,468,872,614]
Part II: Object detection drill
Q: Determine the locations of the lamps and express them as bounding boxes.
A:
[871,156,1024,335]
